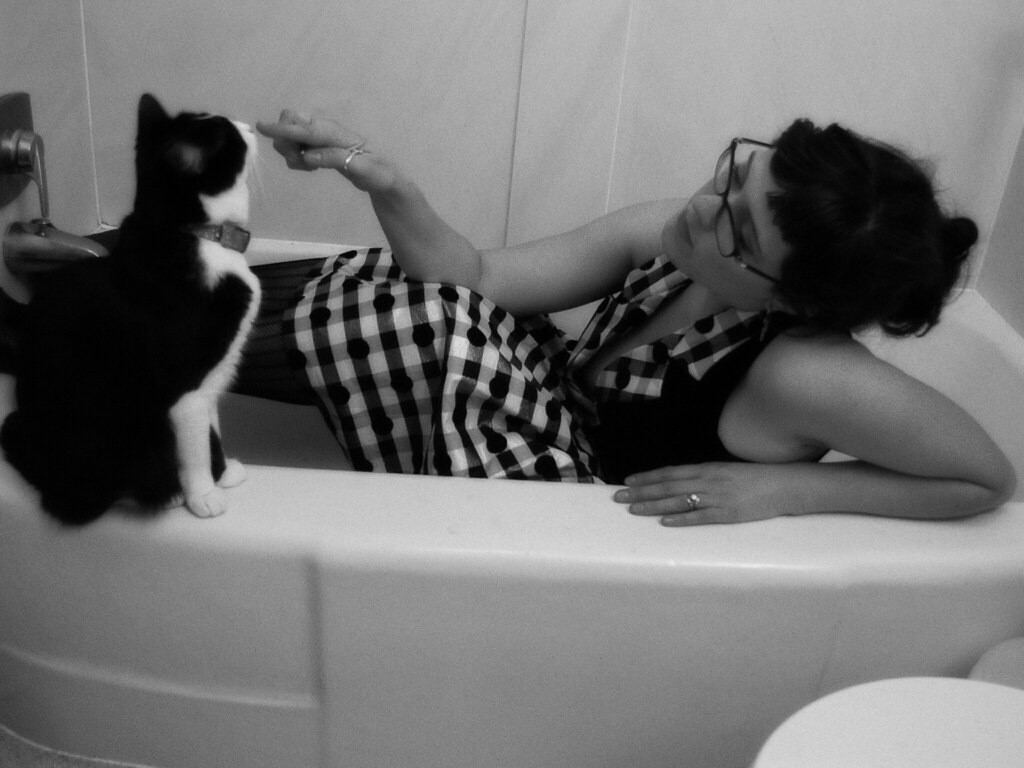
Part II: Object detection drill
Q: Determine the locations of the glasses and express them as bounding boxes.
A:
[714,137,778,286]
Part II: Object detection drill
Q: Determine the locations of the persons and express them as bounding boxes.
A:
[0,118,1017,528]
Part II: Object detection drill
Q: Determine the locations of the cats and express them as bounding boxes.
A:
[1,91,258,525]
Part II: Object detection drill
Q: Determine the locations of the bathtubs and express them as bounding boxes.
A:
[1,233,1022,767]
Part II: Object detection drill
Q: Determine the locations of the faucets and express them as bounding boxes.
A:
[3,220,109,268]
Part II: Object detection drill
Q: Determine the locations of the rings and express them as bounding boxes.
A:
[688,494,701,510]
[344,148,362,177]
